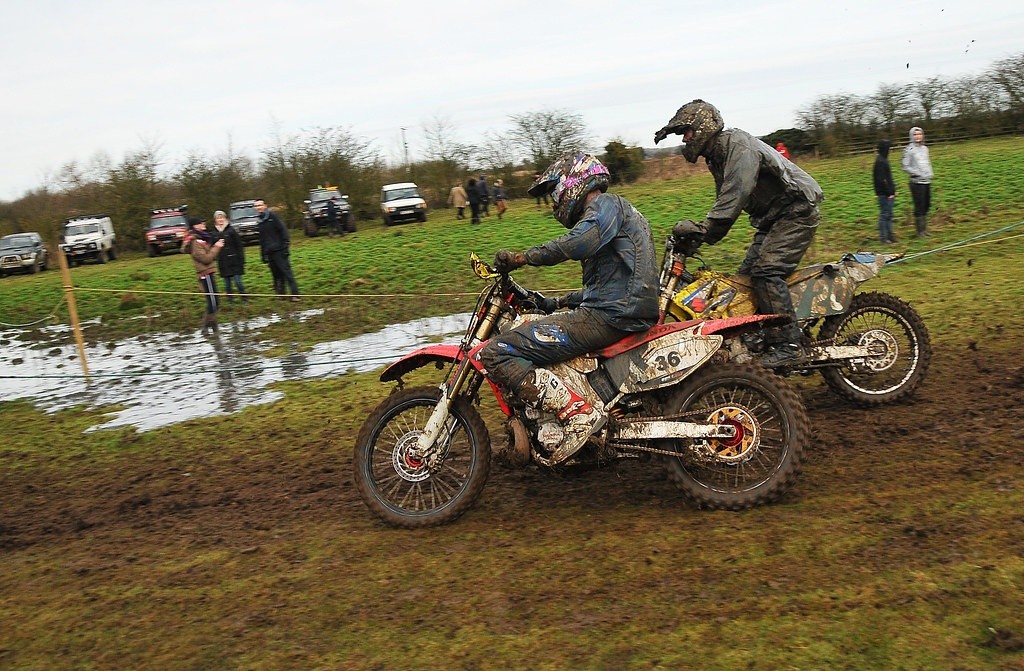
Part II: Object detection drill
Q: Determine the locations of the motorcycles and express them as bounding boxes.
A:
[353,252,813,532]
[650,235,932,407]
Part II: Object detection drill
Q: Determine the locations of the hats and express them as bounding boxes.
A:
[189,216,207,226]
[214,211,227,218]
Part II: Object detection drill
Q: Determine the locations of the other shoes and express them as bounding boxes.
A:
[891,237,900,243]
[275,297,286,299]
[882,240,892,244]
[291,296,300,301]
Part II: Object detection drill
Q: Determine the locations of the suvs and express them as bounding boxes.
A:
[379,183,427,224]
[301,185,359,237]
[228,199,262,244]
[58,215,118,267]
[0,232,50,278]
[145,206,192,256]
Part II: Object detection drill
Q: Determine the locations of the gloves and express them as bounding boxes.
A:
[262,255,269,264]
[496,249,528,270]
[672,219,705,249]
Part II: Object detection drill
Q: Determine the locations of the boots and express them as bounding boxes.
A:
[923,215,934,235]
[520,367,607,465]
[915,216,925,236]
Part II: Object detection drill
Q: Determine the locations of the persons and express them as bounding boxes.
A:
[181,217,225,314]
[776,143,790,159]
[477,174,491,218]
[448,182,468,220]
[873,139,898,244]
[492,179,507,219]
[482,152,658,469]
[209,210,251,302]
[534,172,549,205]
[902,127,934,237]
[327,197,348,237]
[466,179,480,225]
[656,100,824,369]
[253,199,301,302]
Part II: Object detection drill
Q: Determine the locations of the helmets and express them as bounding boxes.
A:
[655,99,724,164]
[528,152,610,228]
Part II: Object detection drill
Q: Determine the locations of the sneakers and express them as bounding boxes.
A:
[752,339,808,367]
